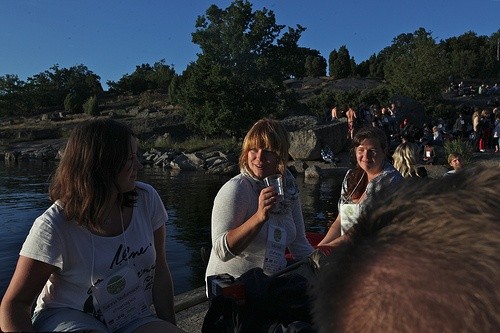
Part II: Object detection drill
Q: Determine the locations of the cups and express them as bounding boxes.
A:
[264,174,284,202]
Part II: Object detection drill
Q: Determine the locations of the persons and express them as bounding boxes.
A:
[200,161,500,333]
[205,119,315,306]
[346,105,356,139]
[393,142,427,178]
[316,126,402,251]
[370,82,500,156]
[331,106,338,122]
[1,117,188,333]
[442,153,469,178]
